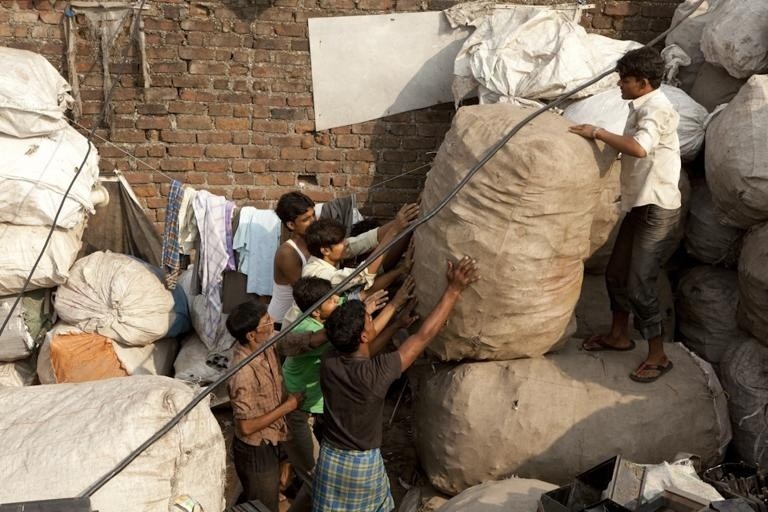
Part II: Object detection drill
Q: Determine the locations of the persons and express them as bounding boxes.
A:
[223,287,390,511]
[274,275,420,511]
[313,253,484,512]
[278,202,422,324]
[568,44,683,383]
[260,191,419,330]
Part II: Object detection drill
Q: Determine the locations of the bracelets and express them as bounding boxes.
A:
[591,125,604,137]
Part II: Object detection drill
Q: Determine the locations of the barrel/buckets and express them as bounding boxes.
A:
[703,463,758,498]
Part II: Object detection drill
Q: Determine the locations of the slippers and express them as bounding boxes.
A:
[583,330,637,352]
[630,359,673,382]
